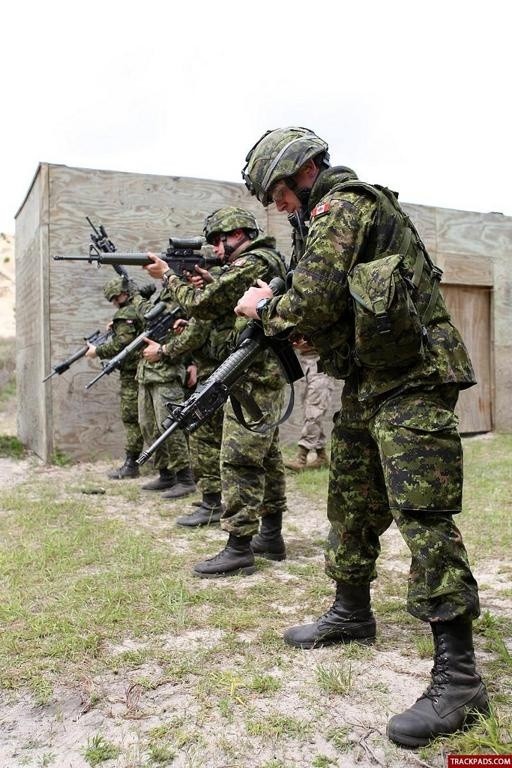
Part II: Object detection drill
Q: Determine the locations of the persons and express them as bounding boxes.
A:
[282,340,336,472]
[85,243,225,530]
[230,124,492,749]
[143,205,288,579]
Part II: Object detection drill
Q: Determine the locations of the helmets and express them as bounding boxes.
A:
[104,277,134,303]
[243,125,329,208]
[202,207,260,242]
[192,244,221,261]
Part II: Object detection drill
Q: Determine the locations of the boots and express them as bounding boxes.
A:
[306,446,329,471]
[283,580,377,649]
[175,489,228,529]
[192,531,257,579]
[107,452,141,479]
[249,511,287,562]
[282,444,310,474]
[139,465,176,492]
[385,610,492,748]
[164,466,198,498]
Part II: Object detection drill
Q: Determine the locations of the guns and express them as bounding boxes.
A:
[135,274,302,464]
[53,236,220,288]
[42,330,117,382]
[86,300,187,391]
[86,217,129,278]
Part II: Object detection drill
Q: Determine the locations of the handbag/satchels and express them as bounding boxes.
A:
[348,247,427,372]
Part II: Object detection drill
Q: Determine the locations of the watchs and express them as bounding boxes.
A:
[255,296,273,319]
